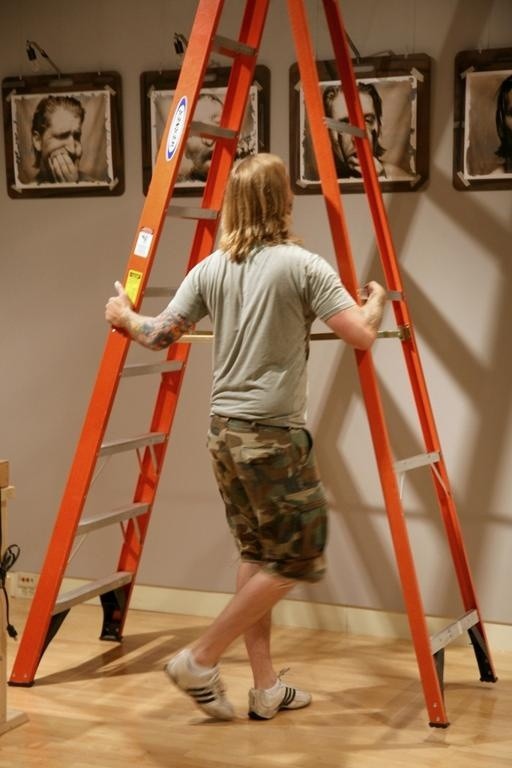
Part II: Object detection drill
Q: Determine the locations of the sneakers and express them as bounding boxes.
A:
[165,648,235,721]
[248,679,311,720]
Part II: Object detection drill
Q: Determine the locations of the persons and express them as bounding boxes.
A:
[177,92,255,180]
[322,78,413,177]
[24,95,99,182]
[484,75,511,174]
[102,152,387,719]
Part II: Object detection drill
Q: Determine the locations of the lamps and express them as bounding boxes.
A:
[26,39,62,73]
[174,33,188,70]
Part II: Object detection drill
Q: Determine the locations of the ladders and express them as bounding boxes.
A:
[8,0,498,728]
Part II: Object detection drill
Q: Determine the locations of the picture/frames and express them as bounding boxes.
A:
[288,53,431,195]
[140,65,270,198]
[2,71,124,199]
[453,48,512,191]
